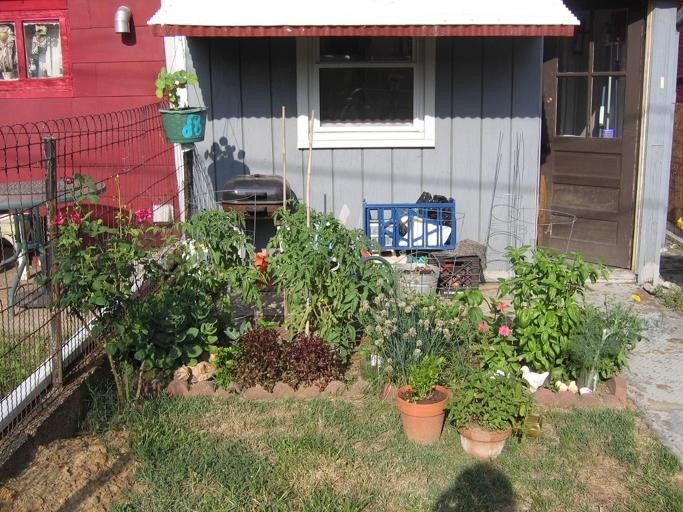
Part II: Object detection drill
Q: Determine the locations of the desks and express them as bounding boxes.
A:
[2,179,106,316]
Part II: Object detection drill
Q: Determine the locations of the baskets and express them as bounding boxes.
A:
[405,252,480,298]
[363,197,456,250]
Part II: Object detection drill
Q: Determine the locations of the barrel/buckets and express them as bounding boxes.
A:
[392,263,440,300]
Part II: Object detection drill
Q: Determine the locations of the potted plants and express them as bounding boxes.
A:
[157,67,208,144]
[393,358,538,461]
